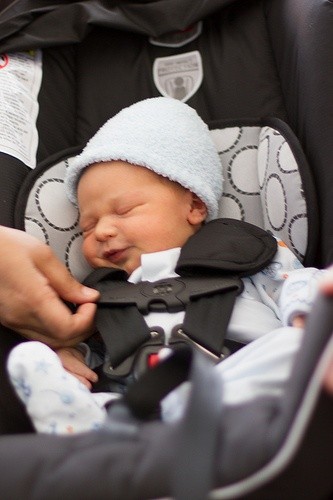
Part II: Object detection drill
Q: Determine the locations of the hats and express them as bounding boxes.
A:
[65,96,224,223]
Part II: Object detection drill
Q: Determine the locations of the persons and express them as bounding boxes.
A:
[10,96,332,438]
[0,224,99,349]
